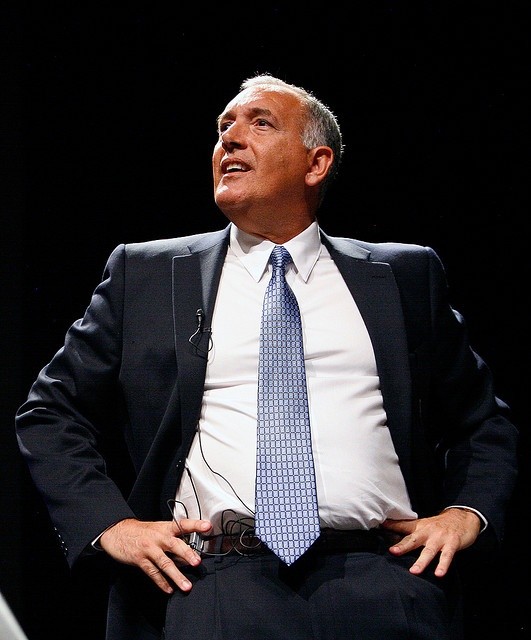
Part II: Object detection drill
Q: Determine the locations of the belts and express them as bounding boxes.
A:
[178,529,382,556]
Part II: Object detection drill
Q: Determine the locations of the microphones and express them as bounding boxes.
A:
[194,308,205,328]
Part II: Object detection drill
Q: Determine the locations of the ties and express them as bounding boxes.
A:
[255,246,320,565]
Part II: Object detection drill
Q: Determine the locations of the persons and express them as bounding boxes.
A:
[15,73,521,640]
